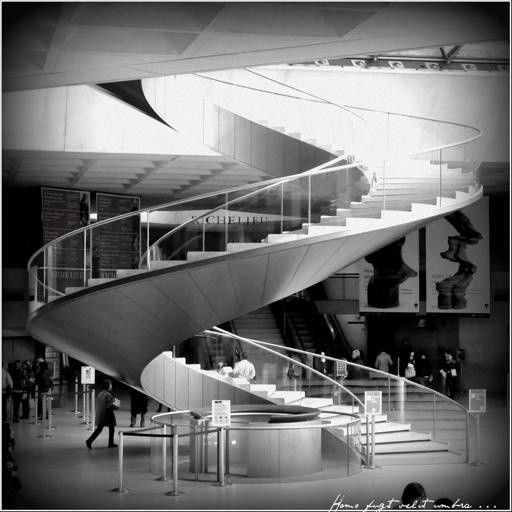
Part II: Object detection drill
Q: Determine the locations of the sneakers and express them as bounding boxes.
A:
[108,443,118,448]
[85,440,92,450]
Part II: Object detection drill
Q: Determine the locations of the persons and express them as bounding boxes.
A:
[156,401,173,414]
[129,386,148,428]
[328,358,348,396]
[2,421,23,509]
[418,354,434,396]
[347,350,365,405]
[442,351,460,400]
[233,350,256,383]
[389,481,428,510]
[289,353,303,390]
[314,351,330,396]
[403,351,417,395]
[3,354,56,425]
[83,378,120,449]
[438,349,451,397]
[432,496,457,509]
[374,348,393,396]
